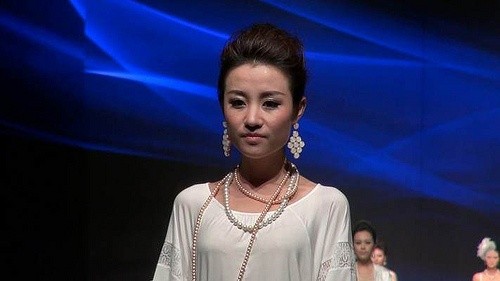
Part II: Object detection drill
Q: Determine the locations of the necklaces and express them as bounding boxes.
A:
[233,162,300,206]
[224,168,296,233]
[192,171,292,280]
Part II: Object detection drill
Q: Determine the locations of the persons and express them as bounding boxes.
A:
[369,241,389,267]
[148,21,357,280]
[349,221,397,280]
[472,237,500,281]
